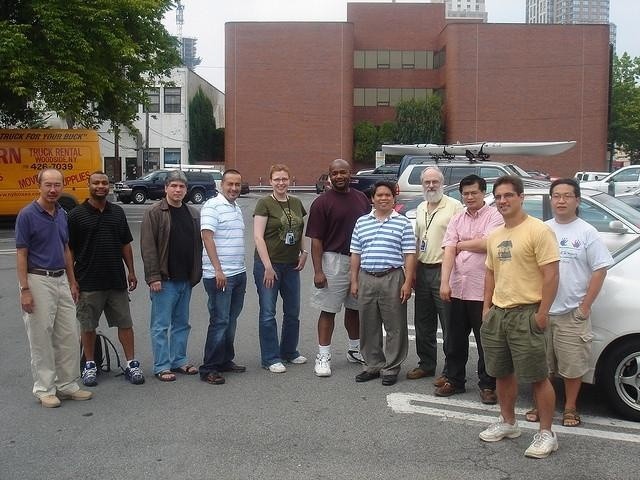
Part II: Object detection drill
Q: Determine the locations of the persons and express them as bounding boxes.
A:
[67,170,144,385]
[305,159,372,377]
[140,170,203,381]
[407,166,463,388]
[253,162,307,373]
[479,174,558,459]
[14,168,92,408]
[199,167,247,384]
[350,181,417,386]
[434,174,506,405]
[525,179,615,424]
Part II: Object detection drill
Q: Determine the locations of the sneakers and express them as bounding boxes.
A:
[291,355,308,364]
[124,360,146,385]
[346,347,366,365]
[82,361,99,386]
[525,431,560,459]
[314,353,333,377]
[40,394,61,408]
[481,414,522,442]
[266,361,287,373]
[58,387,94,400]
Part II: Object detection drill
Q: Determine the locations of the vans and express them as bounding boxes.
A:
[574,170,611,183]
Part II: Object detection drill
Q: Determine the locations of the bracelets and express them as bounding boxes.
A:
[301,249,309,255]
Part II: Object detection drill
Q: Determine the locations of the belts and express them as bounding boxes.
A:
[366,268,398,277]
[27,268,65,278]
[418,261,443,269]
[326,250,352,256]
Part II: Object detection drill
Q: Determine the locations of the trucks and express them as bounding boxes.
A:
[1,128,103,216]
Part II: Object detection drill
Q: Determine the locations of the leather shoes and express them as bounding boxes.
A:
[480,388,497,404]
[436,380,468,397]
[225,364,247,373]
[206,373,226,385]
[382,374,397,386]
[408,367,433,379]
[432,376,448,387]
[356,371,381,381]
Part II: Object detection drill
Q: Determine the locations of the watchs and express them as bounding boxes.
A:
[20,287,29,292]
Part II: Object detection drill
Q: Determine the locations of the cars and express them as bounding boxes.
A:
[315,174,332,194]
[394,179,550,222]
[208,170,249,196]
[348,163,400,205]
[525,169,562,182]
[582,235,640,421]
[483,189,640,257]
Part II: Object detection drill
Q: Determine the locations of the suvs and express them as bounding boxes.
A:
[579,165,640,195]
[396,162,517,207]
[397,156,531,180]
[114,170,217,205]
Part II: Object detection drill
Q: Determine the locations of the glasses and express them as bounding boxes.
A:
[495,192,519,199]
[463,191,485,196]
[271,178,290,183]
[553,193,576,200]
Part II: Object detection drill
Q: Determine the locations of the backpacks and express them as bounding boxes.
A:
[79,330,127,378]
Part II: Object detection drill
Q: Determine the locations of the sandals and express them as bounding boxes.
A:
[157,369,177,382]
[562,408,581,427]
[527,407,541,422]
[172,363,198,376]
